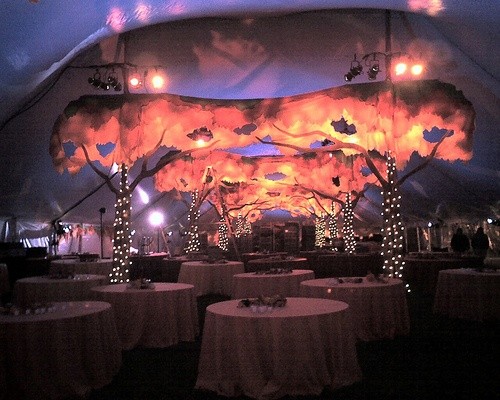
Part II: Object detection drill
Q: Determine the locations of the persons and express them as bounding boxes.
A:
[472,227,489,257]
[451,228,470,255]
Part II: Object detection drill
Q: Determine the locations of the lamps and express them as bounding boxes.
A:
[368,54,380,80]
[344,54,363,82]
[87,69,109,91]
[108,71,122,92]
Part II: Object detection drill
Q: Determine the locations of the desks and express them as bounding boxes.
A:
[231,270,314,299]
[14,273,106,302]
[300,275,410,375]
[51,259,114,287]
[195,300,362,400]
[0,301,118,400]
[433,268,500,330]
[89,280,198,350]
[128,252,168,282]
[242,251,288,274]
[246,257,310,274]
[317,254,381,276]
[177,260,245,299]
[395,256,470,299]
[162,255,211,284]
